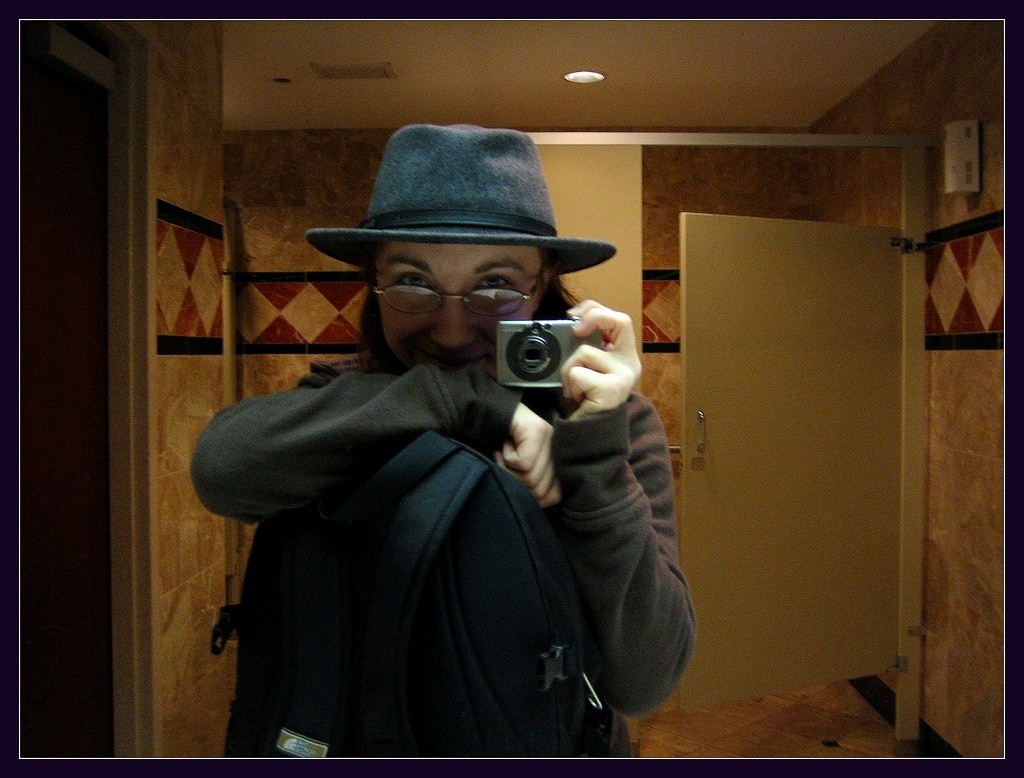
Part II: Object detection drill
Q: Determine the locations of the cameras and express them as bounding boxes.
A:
[496,316,600,388]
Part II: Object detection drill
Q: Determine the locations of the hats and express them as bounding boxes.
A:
[305,124,617,275]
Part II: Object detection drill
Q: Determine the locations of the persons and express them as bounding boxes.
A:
[185,118,696,759]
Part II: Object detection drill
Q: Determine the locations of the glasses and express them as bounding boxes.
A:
[373,276,542,318]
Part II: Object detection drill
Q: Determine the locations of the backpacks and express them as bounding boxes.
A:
[211,429,634,760]
